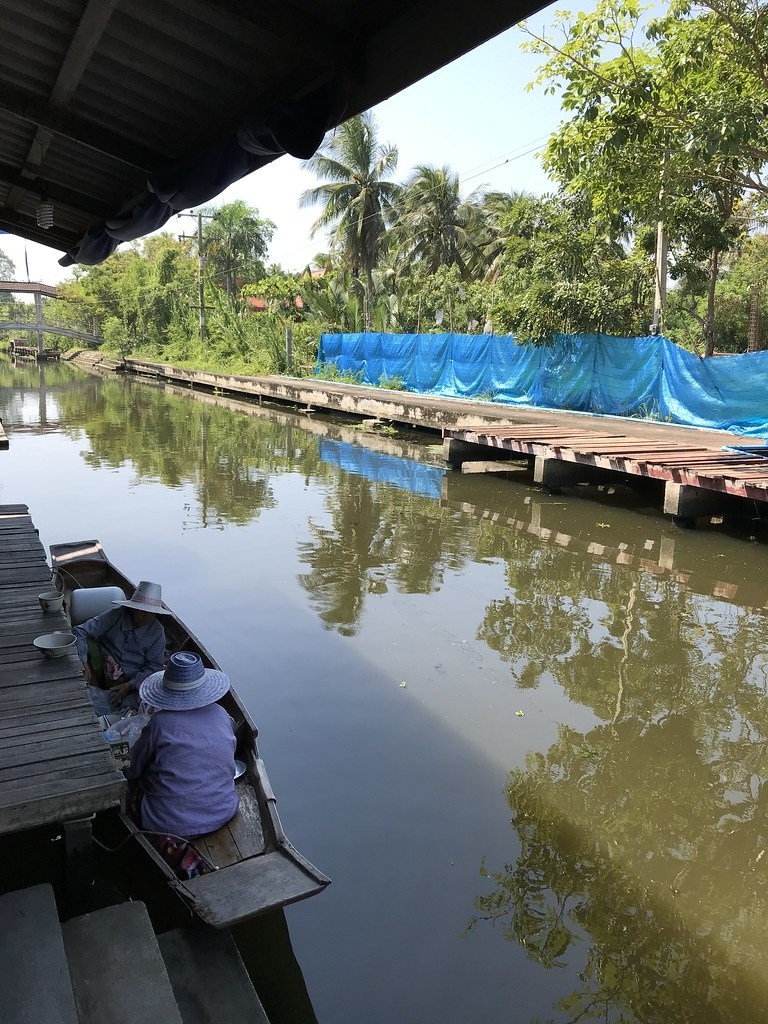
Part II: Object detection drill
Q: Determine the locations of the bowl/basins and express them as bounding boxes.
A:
[98,715,123,732]
[38,591,64,613]
[33,633,77,657]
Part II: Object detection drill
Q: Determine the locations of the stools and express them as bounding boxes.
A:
[135,781,266,878]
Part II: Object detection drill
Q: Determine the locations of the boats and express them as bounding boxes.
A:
[45,539,332,930]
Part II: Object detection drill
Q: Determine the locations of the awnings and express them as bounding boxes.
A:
[0,0,555,253]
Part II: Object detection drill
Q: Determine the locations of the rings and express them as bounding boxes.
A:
[119,700,121,702]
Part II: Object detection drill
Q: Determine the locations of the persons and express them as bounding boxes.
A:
[73,581,172,709]
[125,651,239,837]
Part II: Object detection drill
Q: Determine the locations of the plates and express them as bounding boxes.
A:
[233,760,246,784]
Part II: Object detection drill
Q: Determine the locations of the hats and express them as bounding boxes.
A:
[138,651,230,711]
[110,581,172,614]
